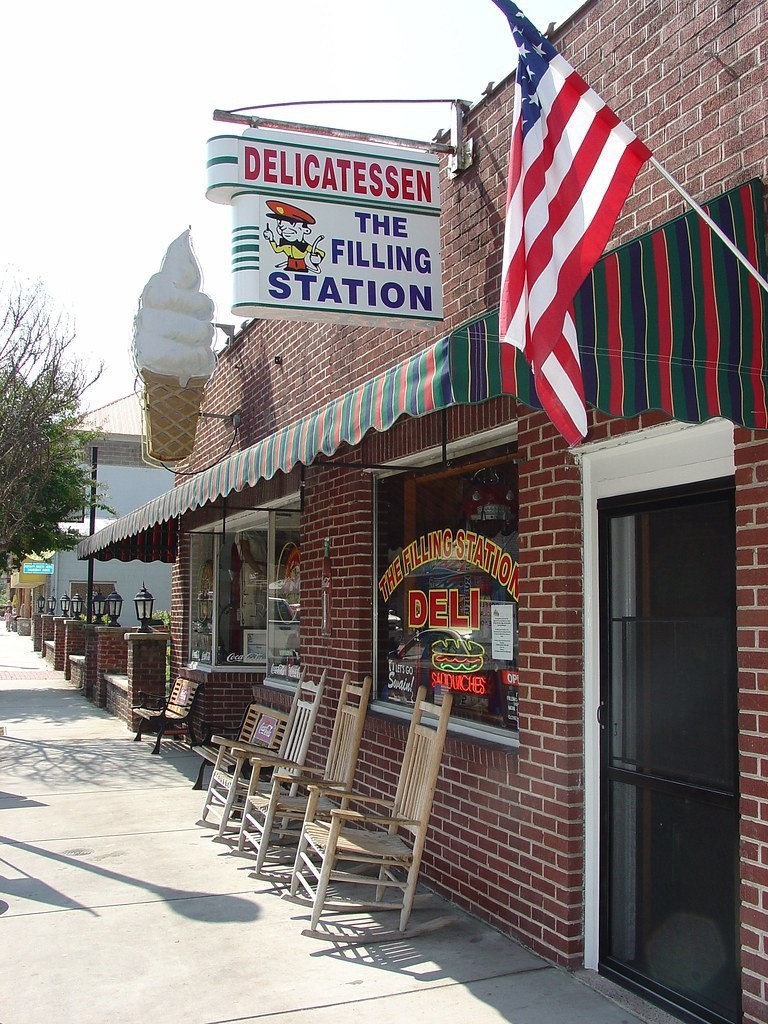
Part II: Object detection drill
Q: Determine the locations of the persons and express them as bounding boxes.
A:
[481,516,519,604]
[466,592,502,716]
[4,609,12,632]
[280,633,300,666]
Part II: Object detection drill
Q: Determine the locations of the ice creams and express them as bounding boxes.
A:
[131,228,215,461]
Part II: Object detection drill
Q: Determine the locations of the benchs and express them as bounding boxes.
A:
[130,676,203,754]
[188,701,290,821]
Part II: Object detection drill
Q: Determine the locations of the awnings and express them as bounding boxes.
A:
[10,571,46,589]
[75,177,768,563]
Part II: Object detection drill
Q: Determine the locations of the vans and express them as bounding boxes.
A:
[198,592,297,626]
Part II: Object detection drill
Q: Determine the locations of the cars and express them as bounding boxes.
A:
[390,630,516,714]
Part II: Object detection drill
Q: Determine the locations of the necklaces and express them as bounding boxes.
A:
[500,532,516,551]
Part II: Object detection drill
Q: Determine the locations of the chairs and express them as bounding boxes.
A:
[212,666,328,864]
[231,672,378,885]
[281,686,459,943]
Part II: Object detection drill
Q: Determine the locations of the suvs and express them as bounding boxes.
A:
[389,609,403,658]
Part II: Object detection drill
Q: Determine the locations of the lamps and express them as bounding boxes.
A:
[37,594,44,613]
[92,586,124,626]
[194,584,214,635]
[59,589,70,617]
[70,589,84,620]
[132,581,154,633]
[47,595,56,615]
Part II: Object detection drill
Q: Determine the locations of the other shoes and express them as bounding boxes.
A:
[8,630,10,632]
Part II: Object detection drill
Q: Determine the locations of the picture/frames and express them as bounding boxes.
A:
[240,626,267,665]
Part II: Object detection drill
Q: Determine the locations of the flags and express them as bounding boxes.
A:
[492,0,654,446]
